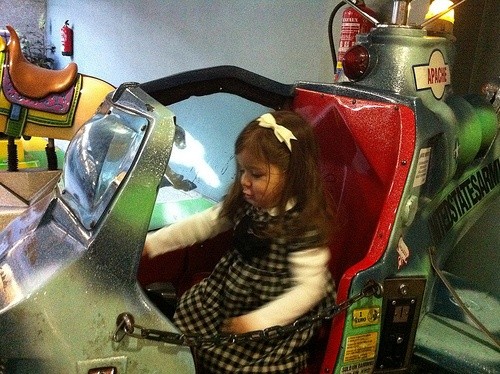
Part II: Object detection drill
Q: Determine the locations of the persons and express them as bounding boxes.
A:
[136,111,337,374]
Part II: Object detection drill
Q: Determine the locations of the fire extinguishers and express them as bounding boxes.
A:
[328,1,378,85]
[60,20,73,56]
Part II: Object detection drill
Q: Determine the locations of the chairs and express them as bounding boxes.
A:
[147,88,416,374]
